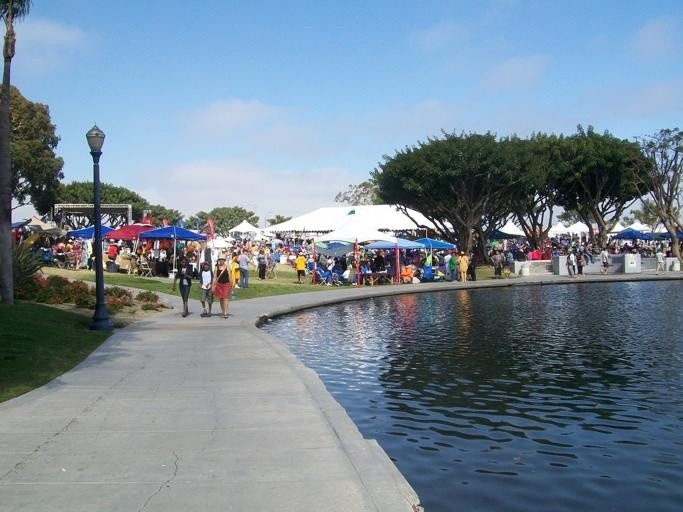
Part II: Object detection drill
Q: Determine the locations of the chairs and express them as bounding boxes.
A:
[139,261,153,277]
[316,271,327,286]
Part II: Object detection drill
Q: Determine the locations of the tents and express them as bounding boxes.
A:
[65,224,112,239]
[414,237,457,248]
[626,222,652,231]
[660,228,683,238]
[606,222,626,233]
[487,220,523,239]
[307,212,400,287]
[565,220,589,233]
[228,220,260,239]
[104,220,158,242]
[613,227,653,240]
[136,226,207,280]
[364,238,426,249]
[547,222,566,235]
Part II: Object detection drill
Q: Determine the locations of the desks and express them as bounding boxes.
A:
[361,272,395,285]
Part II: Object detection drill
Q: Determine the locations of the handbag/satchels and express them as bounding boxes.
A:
[212,266,229,292]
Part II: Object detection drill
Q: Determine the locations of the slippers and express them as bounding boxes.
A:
[225,313,228,319]
[222,314,224,318]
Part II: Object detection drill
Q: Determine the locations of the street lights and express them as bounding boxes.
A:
[84,122,114,333]
[85,122,110,328]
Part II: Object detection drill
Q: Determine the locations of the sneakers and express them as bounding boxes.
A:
[200,313,206,317]
[183,312,186,317]
[207,312,211,317]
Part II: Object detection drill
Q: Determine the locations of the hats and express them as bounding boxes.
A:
[461,252,464,254]
[299,252,303,256]
[219,255,226,260]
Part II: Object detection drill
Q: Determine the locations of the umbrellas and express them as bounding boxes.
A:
[10,217,32,231]
[24,215,59,234]
[306,241,354,259]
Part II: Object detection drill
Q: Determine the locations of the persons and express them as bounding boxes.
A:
[613,242,654,255]
[257,248,265,279]
[573,260,577,275]
[600,248,610,275]
[655,248,665,275]
[173,256,193,317]
[295,252,307,284]
[503,260,512,279]
[497,239,582,254]
[327,256,340,286]
[56,239,65,254]
[576,250,585,276]
[458,250,470,282]
[449,250,458,282]
[231,237,287,247]
[468,250,477,280]
[339,264,354,282]
[583,243,594,264]
[213,254,236,319]
[239,250,252,289]
[198,261,214,317]
[444,253,452,280]
[307,255,318,272]
[232,250,242,288]
[268,249,278,279]
[490,251,505,279]
[567,249,574,278]
[374,250,384,271]
[108,245,118,261]
[65,239,75,270]
